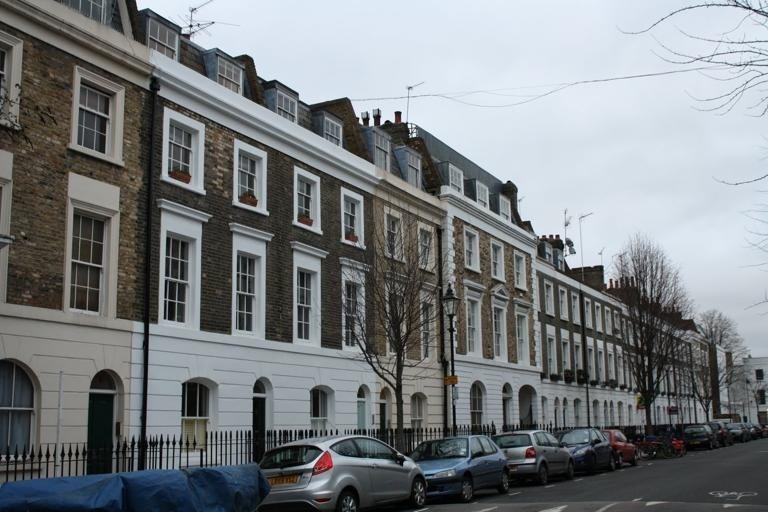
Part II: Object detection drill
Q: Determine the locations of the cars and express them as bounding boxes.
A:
[684,421,768,450]
[408,434,510,502]
[552,427,638,476]
[252,434,428,511]
[490,430,575,484]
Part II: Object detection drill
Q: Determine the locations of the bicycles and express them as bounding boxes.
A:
[634,431,688,460]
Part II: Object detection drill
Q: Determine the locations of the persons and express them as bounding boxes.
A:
[625,430,648,442]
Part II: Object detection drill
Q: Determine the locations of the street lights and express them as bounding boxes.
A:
[441,283,462,436]
